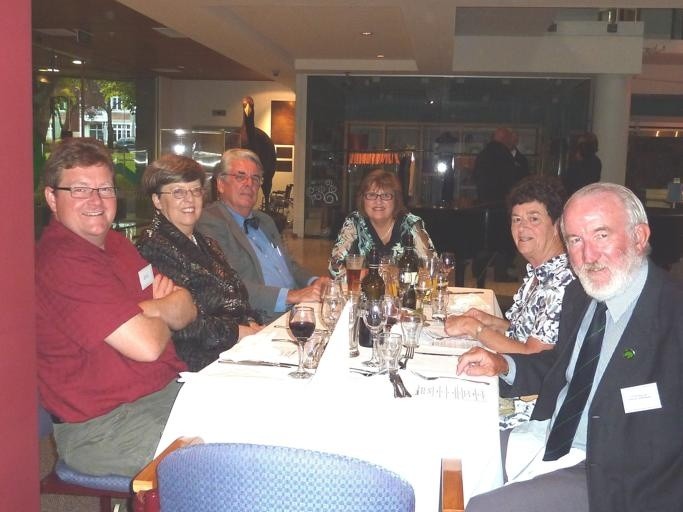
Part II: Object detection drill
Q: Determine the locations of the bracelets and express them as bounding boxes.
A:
[474,324,485,340]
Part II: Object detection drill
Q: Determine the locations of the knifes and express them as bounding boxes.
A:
[394,371,411,397]
[389,371,404,398]
[415,352,462,356]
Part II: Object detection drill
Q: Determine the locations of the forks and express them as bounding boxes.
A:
[424,328,478,341]
[413,370,490,386]
[399,346,414,370]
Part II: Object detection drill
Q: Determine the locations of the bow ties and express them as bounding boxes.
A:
[243,217,260,234]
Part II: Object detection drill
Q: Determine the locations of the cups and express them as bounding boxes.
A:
[400,311,423,348]
[438,252,455,291]
[340,290,362,357]
[287,306,315,379]
[361,299,388,366]
[320,299,342,336]
[430,289,449,320]
[376,332,402,375]
[319,280,341,303]
[343,255,365,295]
[413,273,432,309]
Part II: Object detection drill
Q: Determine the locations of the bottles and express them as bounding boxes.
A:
[398,247,419,309]
[358,250,386,349]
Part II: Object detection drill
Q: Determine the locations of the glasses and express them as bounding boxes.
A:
[155,188,203,199]
[365,192,392,201]
[220,172,261,185]
[54,186,117,199]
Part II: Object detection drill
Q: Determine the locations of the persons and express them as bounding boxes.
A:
[445,174,579,432]
[328,169,441,284]
[133,153,267,372]
[35,137,197,477]
[456,182,682,512]
[472,125,520,282]
[194,148,333,325]
[563,134,601,197]
[506,128,531,181]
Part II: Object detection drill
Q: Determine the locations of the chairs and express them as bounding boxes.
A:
[36,400,133,509]
[131,432,466,512]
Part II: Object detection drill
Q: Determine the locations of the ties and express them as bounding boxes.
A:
[542,301,608,461]
